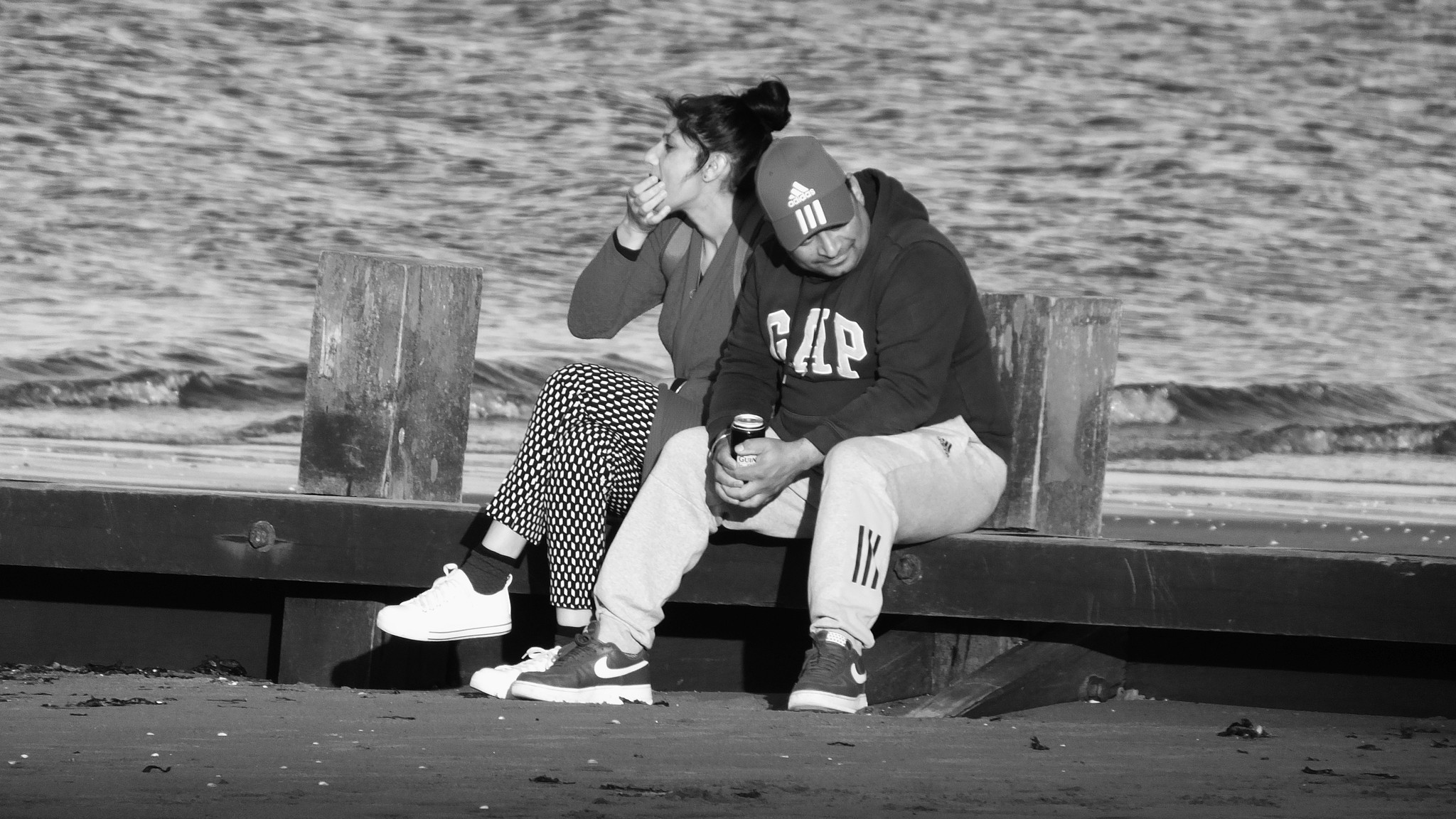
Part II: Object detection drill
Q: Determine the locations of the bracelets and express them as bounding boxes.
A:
[709,432,730,462]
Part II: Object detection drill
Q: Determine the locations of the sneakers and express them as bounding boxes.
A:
[375,563,513,641]
[511,621,654,706]
[787,631,869,713]
[468,644,562,700]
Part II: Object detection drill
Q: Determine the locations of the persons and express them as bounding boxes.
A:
[507,138,1015,714]
[375,77,793,699]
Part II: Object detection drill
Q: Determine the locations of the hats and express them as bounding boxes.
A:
[755,134,855,251]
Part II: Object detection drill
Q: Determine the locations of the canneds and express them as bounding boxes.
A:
[730,413,765,467]
